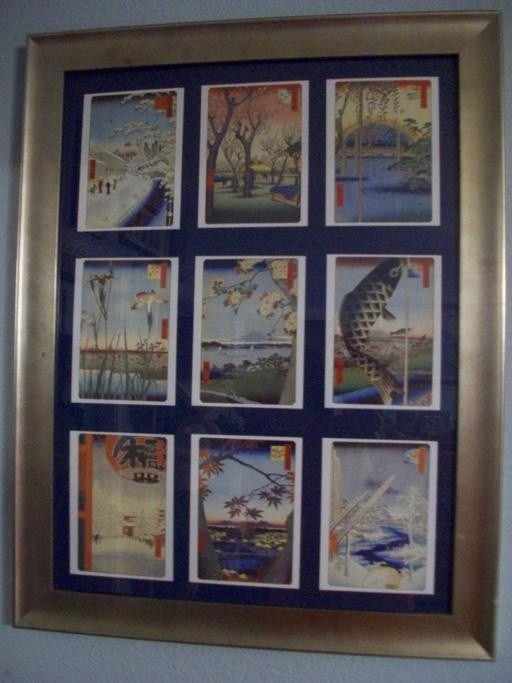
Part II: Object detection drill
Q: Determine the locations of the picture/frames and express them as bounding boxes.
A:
[9,10,506,660]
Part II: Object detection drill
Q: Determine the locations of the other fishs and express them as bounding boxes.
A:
[337,254,408,407]
